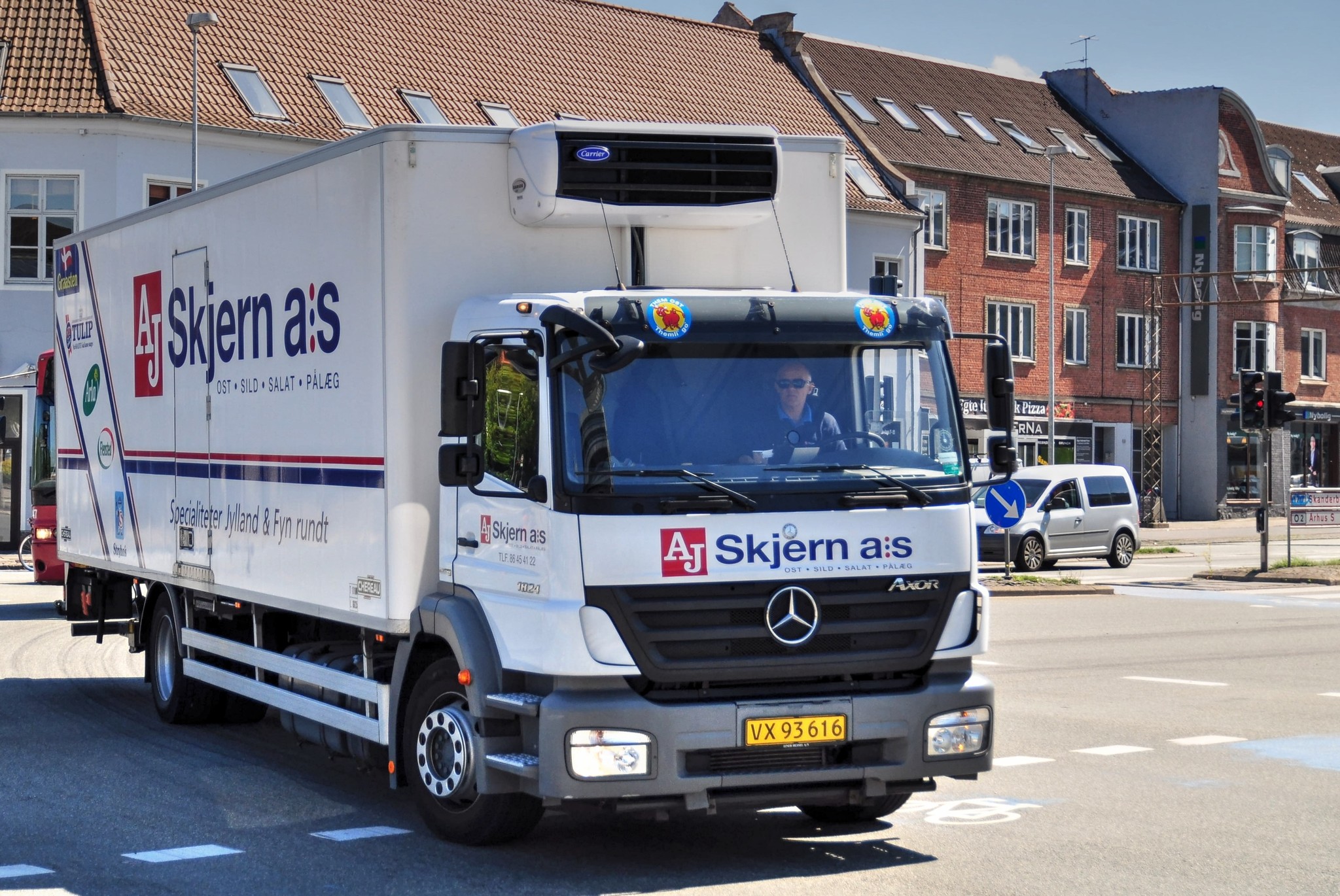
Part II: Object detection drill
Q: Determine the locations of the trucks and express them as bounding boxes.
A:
[50,118,1021,847]
[0,349,66,586]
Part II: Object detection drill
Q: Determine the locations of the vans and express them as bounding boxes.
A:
[971,463,1142,572]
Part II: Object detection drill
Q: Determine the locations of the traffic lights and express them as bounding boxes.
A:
[1264,370,1296,428]
[881,275,904,297]
[1228,393,1241,422]
[1238,367,1265,433]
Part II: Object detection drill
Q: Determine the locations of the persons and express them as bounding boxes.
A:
[724,359,849,467]
[1041,484,1073,511]
[1302,434,1322,489]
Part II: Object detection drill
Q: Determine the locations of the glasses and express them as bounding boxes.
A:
[776,379,812,388]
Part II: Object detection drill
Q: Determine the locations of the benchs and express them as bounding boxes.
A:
[1225,498,1274,518]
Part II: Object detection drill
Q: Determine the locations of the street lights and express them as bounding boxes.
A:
[184,11,218,192]
